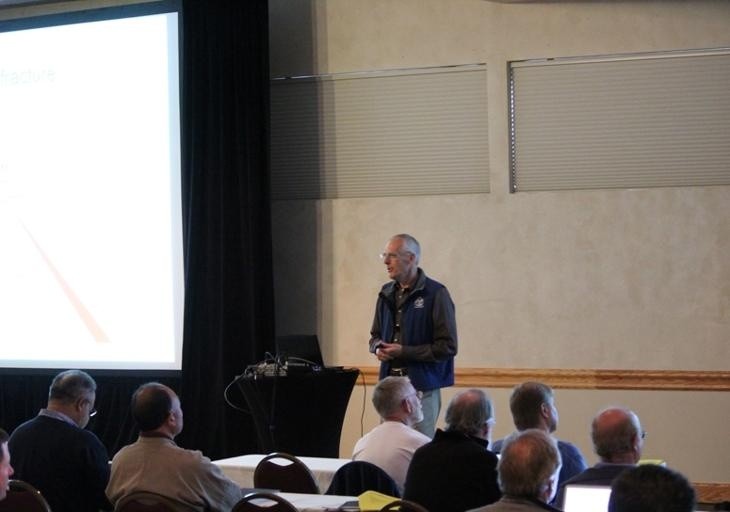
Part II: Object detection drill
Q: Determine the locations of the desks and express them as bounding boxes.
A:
[248,489,357,512]
[212,454,354,492]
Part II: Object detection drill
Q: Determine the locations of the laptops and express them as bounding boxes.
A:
[277,335,343,371]
[561,483,612,512]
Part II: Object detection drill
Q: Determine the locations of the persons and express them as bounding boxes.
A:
[400,388,504,511]
[105,383,244,511]
[607,464,698,511]
[366,233,458,441]
[8,369,110,509]
[351,376,432,501]
[555,407,649,512]
[468,429,564,511]
[1,428,16,501]
[487,381,587,506]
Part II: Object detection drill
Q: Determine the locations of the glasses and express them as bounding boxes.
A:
[85,399,97,416]
[406,390,423,400]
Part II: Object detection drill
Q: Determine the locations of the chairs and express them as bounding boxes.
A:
[6,479,51,511]
[252,451,319,493]
[115,490,176,511]
[231,494,297,512]
[380,500,429,512]
[327,462,403,498]
